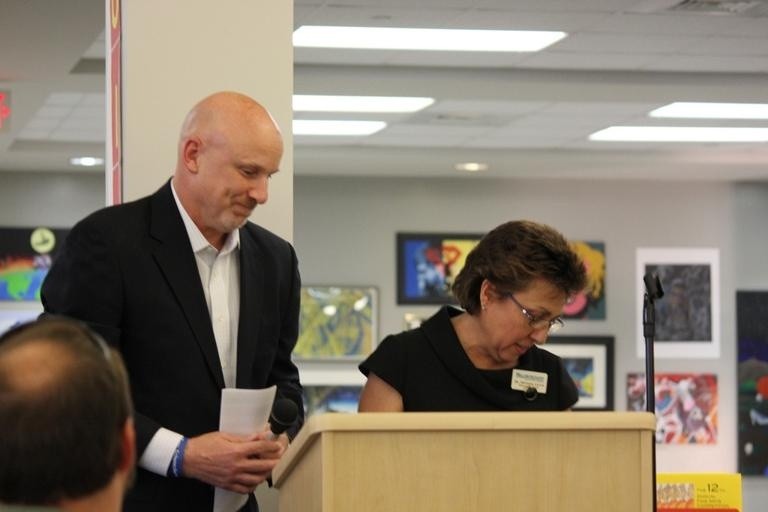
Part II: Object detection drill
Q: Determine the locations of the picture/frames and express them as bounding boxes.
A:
[396,232,488,306]
[292,283,379,362]
[539,334,615,411]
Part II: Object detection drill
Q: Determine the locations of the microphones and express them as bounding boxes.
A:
[248,399,298,460]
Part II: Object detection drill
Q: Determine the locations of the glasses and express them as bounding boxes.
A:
[508,293,564,336]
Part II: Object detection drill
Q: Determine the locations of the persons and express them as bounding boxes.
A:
[358,219,588,412]
[0,313,136,512]
[41,90,305,512]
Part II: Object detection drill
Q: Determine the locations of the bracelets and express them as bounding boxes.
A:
[172,438,187,478]
